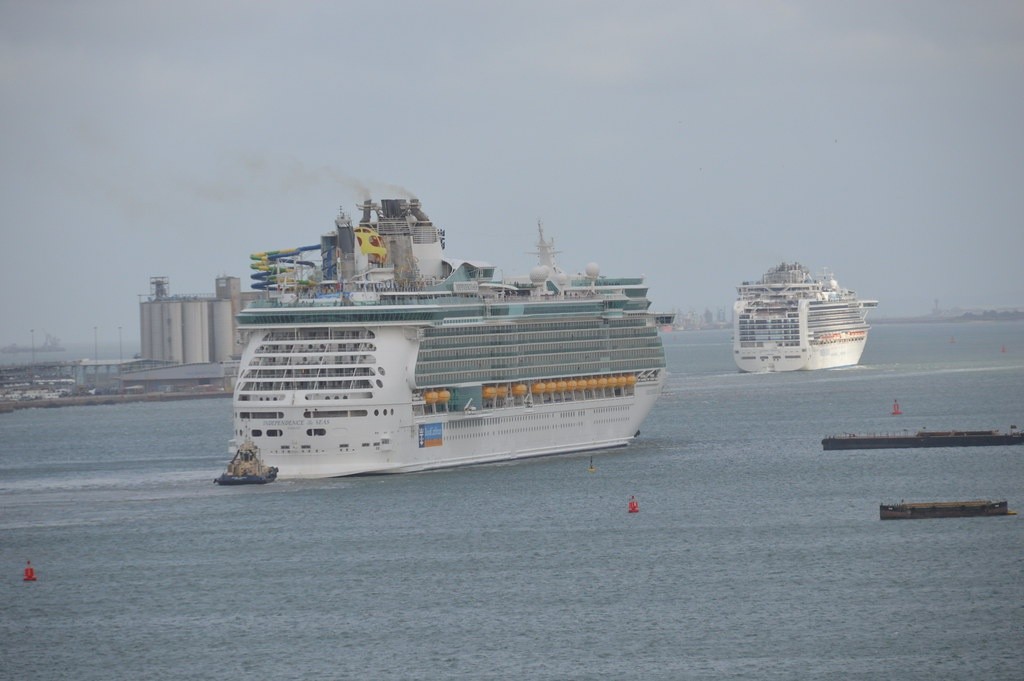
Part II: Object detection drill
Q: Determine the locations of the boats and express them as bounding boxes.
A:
[482,383,527,400]
[531,374,638,394]
[424,389,452,405]
[212,434,279,487]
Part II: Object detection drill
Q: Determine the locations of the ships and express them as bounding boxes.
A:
[732,260,880,373]
[224,195,669,480]
